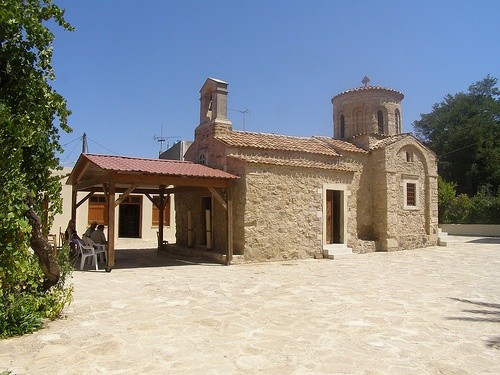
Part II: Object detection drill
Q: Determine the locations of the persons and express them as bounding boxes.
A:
[90,224,108,261]
[64,219,85,256]
[84,220,99,239]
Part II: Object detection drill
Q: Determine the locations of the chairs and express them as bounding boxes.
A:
[48,234,56,256]
[61,234,66,248]
[156,232,168,252]
[82,236,107,266]
[76,239,99,271]
